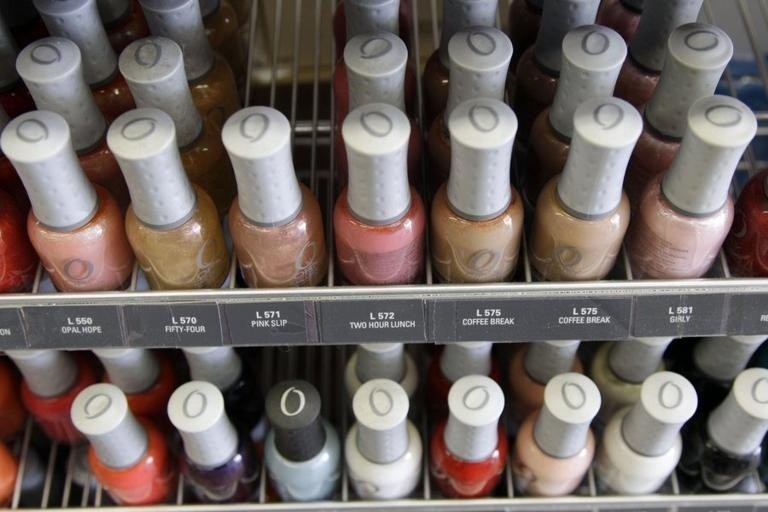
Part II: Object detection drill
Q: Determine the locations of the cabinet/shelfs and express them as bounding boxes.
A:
[0,1,768,512]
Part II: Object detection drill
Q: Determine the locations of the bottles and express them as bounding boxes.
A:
[0,1,766,289]
[2,327,767,511]
